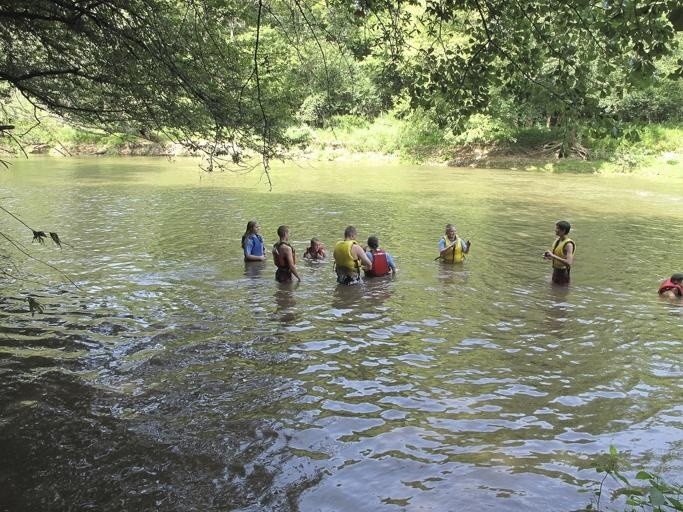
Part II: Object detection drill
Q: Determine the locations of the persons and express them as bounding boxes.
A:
[359,235,395,278]
[437,223,472,262]
[304,237,325,259]
[540,220,574,284]
[241,220,266,260]
[272,225,300,283]
[333,225,371,285]
[659,271,682,300]
